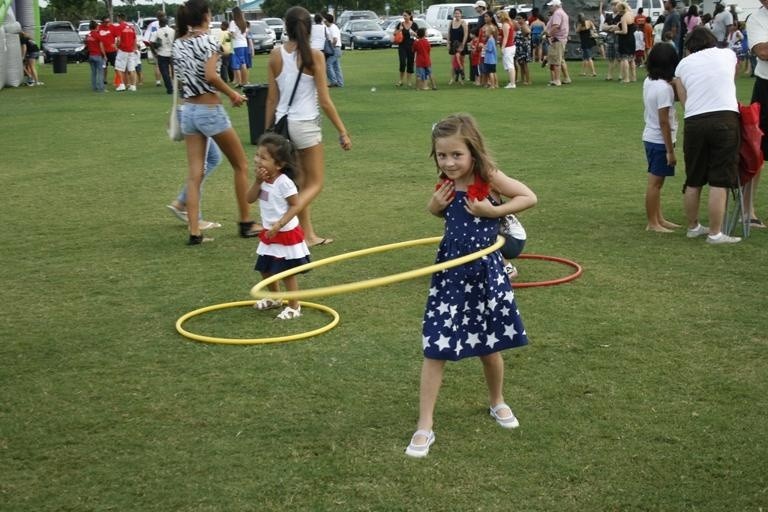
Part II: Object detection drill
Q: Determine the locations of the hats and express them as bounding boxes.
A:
[546,0,561,6]
[471,0,486,9]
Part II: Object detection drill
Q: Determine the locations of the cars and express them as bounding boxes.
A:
[41,16,176,63]
[209,8,443,52]
[493,3,532,41]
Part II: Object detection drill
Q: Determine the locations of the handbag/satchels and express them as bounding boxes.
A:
[166,106,183,141]
[274,114,294,178]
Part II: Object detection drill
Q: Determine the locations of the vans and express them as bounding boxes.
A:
[598,0,665,33]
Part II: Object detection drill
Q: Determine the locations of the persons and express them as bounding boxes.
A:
[674,25,743,245]
[404,111,539,459]
[263,5,354,249]
[395,1,758,92]
[170,0,263,247]
[310,12,346,86]
[736,1,768,228]
[84,6,254,94]
[641,40,683,233]
[246,131,312,321]
[19,31,40,84]
[171,137,223,229]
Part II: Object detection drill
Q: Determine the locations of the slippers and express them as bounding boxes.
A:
[166,204,221,244]
[745,218,767,228]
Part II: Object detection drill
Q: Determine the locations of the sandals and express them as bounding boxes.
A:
[239,220,262,237]
[253,297,282,312]
[274,303,302,319]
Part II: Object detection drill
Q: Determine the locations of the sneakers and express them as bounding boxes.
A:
[547,78,572,86]
[687,223,709,238]
[113,81,143,92]
[490,404,520,428]
[506,266,518,278]
[504,82,516,88]
[404,429,435,457]
[705,232,742,243]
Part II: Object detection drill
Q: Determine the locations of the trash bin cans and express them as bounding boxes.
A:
[243,84,268,145]
[53,52,67,73]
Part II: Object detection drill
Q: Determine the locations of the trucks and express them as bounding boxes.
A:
[425,3,483,40]
